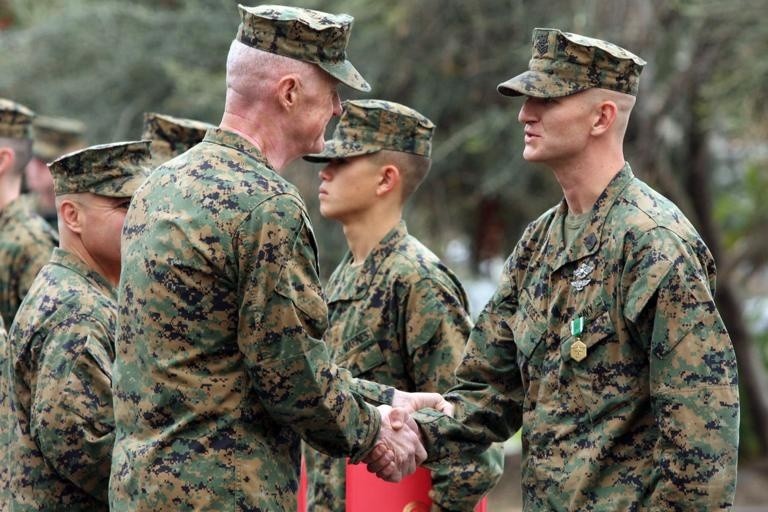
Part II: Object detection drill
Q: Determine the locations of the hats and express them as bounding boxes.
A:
[47,141,153,197]
[1,99,36,137]
[32,118,88,162]
[303,100,436,162]
[235,4,371,93]
[497,28,646,97]
[141,112,216,163]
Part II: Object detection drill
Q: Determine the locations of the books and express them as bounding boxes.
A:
[296,449,487,512]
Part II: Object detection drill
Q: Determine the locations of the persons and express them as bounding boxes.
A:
[0,99,218,511]
[362,25,744,511]
[299,97,508,512]
[105,1,459,510]
